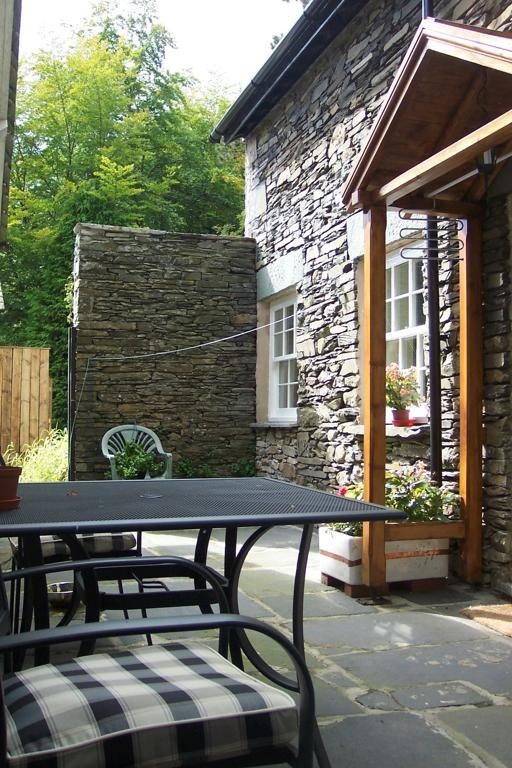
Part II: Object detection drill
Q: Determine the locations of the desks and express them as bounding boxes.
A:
[1,467,409,766]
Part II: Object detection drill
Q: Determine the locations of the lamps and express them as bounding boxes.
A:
[473,145,498,187]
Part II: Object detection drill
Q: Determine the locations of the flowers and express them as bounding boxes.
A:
[384,359,422,407]
[318,460,461,542]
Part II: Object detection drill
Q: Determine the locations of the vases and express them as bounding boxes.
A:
[391,408,412,424]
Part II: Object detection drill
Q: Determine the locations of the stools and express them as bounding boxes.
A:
[2,612,310,766]
[2,532,159,655]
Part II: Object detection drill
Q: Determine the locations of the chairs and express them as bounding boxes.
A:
[99,425,179,480]
